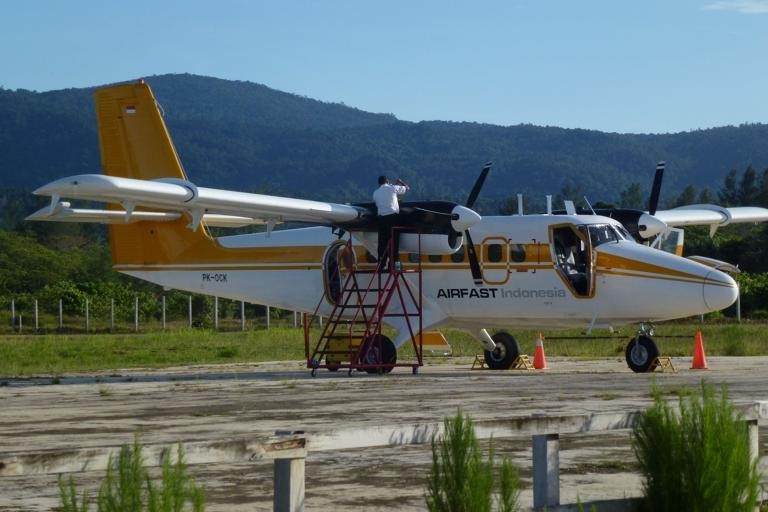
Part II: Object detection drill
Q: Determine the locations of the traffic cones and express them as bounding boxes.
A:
[690,328,709,369]
[531,331,549,370]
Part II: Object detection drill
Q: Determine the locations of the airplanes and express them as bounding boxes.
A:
[24,79,767,379]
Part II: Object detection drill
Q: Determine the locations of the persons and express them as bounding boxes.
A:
[373,173,409,267]
